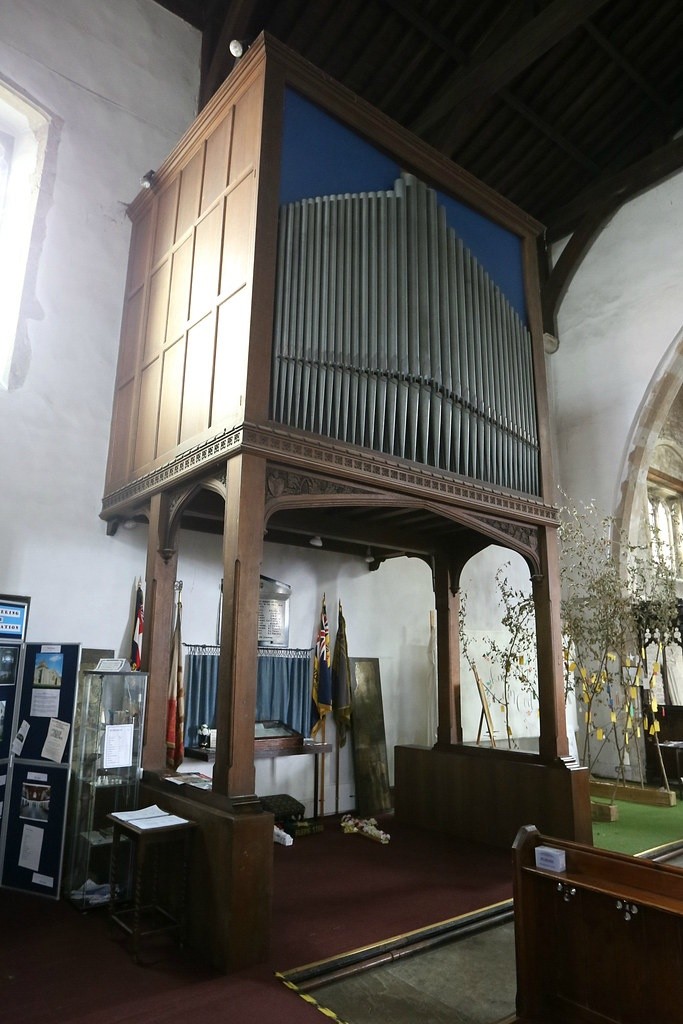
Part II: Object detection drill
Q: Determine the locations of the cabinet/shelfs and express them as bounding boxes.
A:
[64,669,150,916]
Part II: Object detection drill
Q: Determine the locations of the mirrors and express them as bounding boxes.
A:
[348,655,393,818]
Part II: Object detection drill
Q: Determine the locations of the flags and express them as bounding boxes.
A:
[311,603,332,736]
[168,602,186,772]
[334,606,353,748]
[131,587,145,670]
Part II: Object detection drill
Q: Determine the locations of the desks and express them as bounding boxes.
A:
[184,743,333,837]
[655,742,683,801]
[107,806,201,966]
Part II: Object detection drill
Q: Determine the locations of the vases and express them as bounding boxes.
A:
[198,734,210,748]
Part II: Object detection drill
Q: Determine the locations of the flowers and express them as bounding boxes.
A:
[197,723,211,736]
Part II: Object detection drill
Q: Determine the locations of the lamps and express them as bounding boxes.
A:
[123,518,136,529]
[309,535,323,547]
[363,545,374,563]
